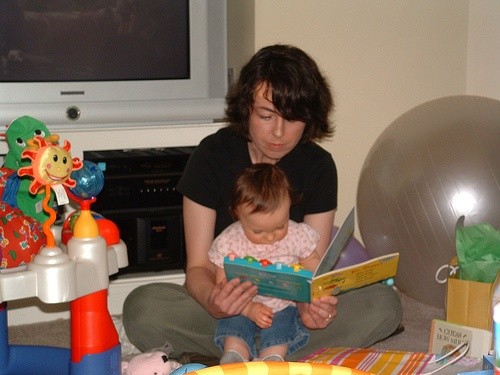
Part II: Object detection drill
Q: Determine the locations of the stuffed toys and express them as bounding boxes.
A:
[120,352,207,375]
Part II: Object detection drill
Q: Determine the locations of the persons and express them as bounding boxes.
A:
[122,43,404,361]
[207,163,321,366]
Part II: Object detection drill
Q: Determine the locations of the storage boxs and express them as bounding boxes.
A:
[445,257,500,332]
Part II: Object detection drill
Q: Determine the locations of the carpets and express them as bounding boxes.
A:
[7,292,446,375]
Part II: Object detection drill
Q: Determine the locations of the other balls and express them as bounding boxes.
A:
[353,91,500,310]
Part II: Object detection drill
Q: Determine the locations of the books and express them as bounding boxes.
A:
[428,318,493,361]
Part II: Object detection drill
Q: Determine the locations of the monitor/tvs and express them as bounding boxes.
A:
[0,0,229,134]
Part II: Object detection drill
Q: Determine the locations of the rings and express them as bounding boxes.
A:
[325,313,332,322]
